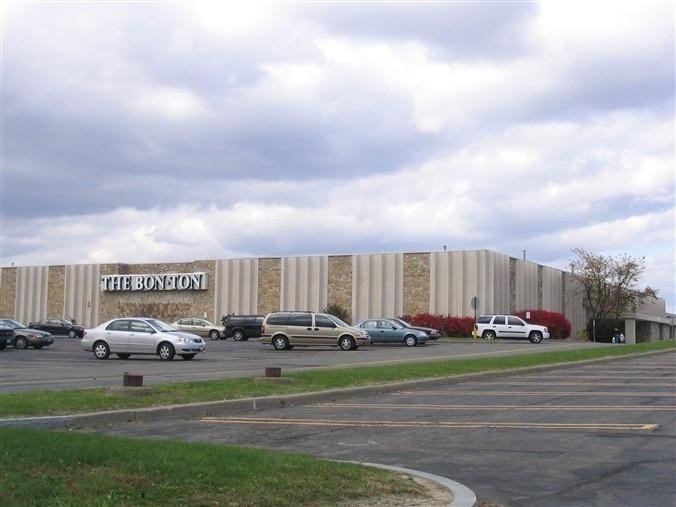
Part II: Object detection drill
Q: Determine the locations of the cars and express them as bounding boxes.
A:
[471,314,550,344]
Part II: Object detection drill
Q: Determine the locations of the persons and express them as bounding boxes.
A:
[611,332,625,343]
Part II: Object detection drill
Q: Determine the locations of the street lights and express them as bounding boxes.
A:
[666,315,676,339]
